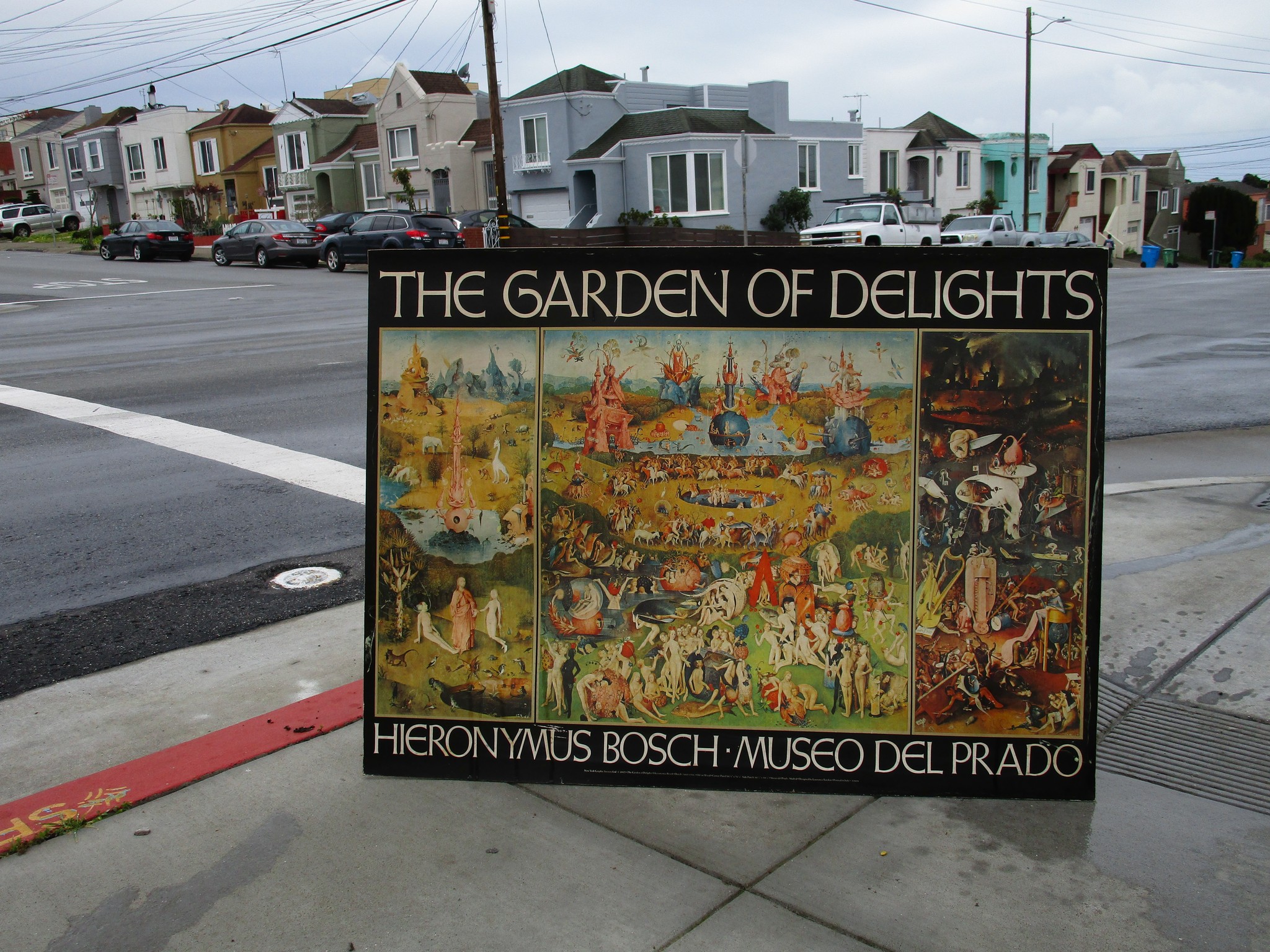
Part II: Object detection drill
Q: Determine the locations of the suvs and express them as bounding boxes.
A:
[298,208,539,273]
[0,203,85,240]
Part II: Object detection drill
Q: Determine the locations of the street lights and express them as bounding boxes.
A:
[166,198,171,221]
[144,200,149,219]
[244,193,250,220]
[151,198,155,219]
[179,196,185,224]
[1023,6,1071,232]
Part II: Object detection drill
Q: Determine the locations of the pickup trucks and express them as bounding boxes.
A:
[940,215,1040,247]
[799,195,941,245]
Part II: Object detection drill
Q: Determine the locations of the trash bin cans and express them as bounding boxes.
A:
[1140,246,1161,268]
[1207,250,1222,268]
[1162,248,1180,268]
[1229,251,1244,268]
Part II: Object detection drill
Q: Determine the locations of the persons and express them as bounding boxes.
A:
[1103,234,1115,268]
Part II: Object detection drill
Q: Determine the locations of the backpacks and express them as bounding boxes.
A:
[1106,240,1114,249]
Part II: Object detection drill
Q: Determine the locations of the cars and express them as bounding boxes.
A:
[99,220,195,262]
[212,220,322,269]
[1039,233,1096,248]
